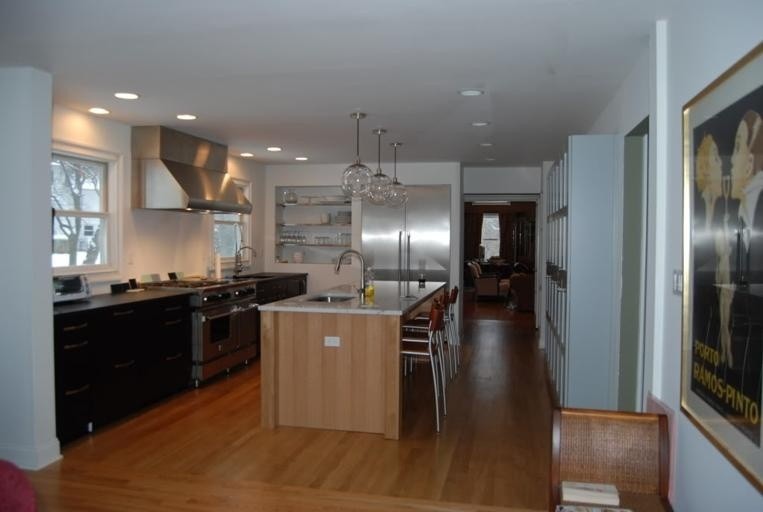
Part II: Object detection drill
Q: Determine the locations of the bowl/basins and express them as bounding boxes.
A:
[321,194,345,201]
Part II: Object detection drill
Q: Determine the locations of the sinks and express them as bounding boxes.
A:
[221,275,275,280]
[296,292,358,304]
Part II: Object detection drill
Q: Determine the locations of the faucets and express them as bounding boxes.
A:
[335,249,365,305]
[234,246,256,277]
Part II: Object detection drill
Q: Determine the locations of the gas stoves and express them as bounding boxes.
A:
[141,279,255,307]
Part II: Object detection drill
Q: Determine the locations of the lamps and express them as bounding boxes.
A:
[386,142,409,207]
[340,111,374,199]
[366,129,394,205]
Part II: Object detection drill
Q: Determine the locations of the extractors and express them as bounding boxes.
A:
[131,125,255,215]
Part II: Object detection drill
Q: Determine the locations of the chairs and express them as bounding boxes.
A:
[468,262,508,300]
[471,259,510,282]
[545,408,674,512]
[402,281,462,432]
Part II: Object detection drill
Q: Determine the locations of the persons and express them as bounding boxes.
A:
[715,107,763,391]
[691,133,732,350]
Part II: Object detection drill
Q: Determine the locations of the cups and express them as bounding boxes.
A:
[319,212,330,225]
[417,273,425,284]
[292,250,303,264]
[279,228,350,244]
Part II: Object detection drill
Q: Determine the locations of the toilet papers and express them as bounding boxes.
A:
[214,253,221,274]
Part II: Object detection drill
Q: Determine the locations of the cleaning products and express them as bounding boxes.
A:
[364,267,375,305]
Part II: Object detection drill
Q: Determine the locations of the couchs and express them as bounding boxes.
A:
[510,271,534,313]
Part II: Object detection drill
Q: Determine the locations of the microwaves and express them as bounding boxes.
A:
[51,273,91,304]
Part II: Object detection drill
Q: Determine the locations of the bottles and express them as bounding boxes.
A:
[284,187,297,204]
[364,266,374,296]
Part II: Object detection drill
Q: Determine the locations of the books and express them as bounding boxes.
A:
[553,504,633,511]
[560,479,619,506]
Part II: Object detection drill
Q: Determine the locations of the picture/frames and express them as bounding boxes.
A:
[679,37,761,489]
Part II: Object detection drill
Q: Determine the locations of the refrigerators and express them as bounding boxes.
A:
[360,184,451,283]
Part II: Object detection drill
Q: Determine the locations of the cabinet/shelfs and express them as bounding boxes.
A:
[103,302,161,423]
[52,316,103,445]
[544,135,621,410]
[275,198,350,250]
[161,290,190,404]
[256,273,309,302]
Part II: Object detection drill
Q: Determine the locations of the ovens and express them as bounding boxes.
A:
[191,297,256,388]
